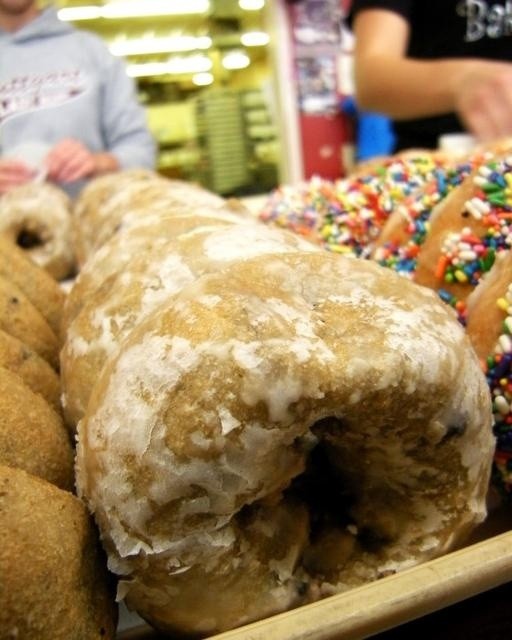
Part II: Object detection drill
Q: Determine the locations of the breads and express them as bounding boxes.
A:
[0,134,512,640]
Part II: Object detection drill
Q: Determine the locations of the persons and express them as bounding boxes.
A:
[0,0,160,196]
[341,0,511,155]
